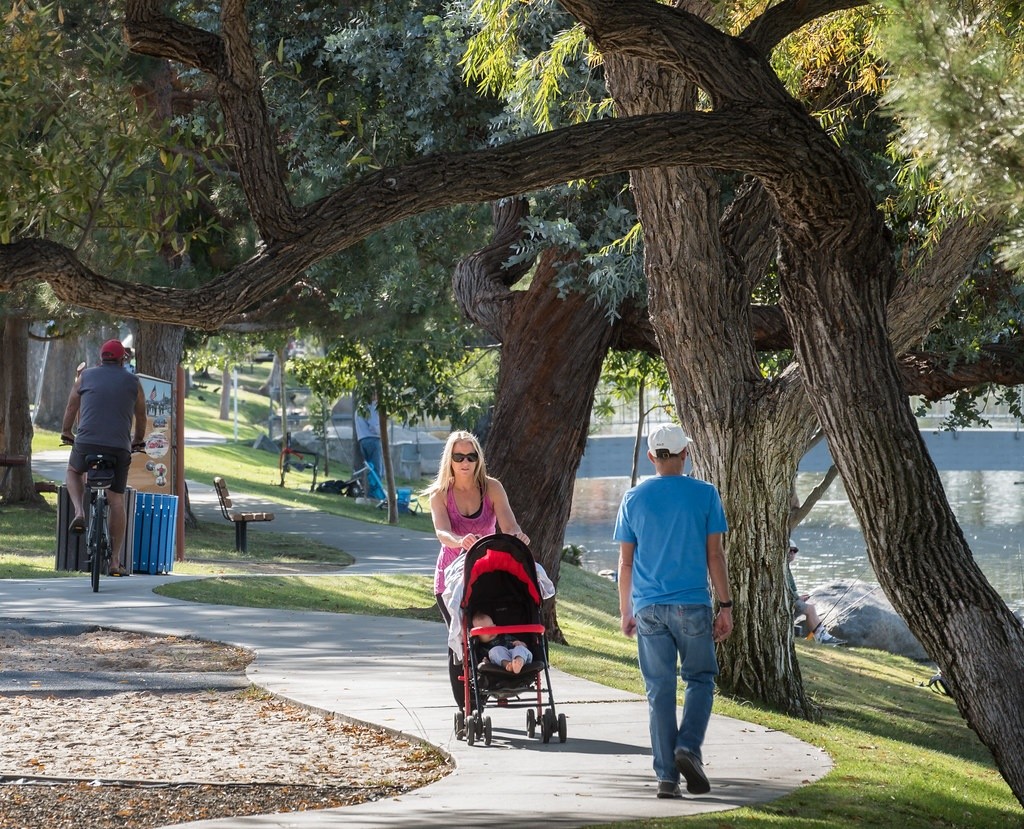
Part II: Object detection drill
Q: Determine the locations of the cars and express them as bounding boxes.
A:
[254,338,309,363]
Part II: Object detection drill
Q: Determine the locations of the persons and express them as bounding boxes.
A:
[156,465,164,475]
[418,430,530,716]
[788,540,848,646]
[612,423,733,797]
[355,389,384,498]
[62,338,147,575]
[472,613,532,673]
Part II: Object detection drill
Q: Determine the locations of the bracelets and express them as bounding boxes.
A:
[719,601,732,608]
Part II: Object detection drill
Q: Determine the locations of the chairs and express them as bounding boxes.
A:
[281,432,320,492]
[352,460,424,515]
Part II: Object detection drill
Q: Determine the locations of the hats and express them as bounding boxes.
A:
[101,339,130,359]
[789,539,797,547]
[647,423,693,458]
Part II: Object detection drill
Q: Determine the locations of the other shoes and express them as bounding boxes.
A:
[68,517,86,536]
[109,563,126,576]
[817,637,848,645]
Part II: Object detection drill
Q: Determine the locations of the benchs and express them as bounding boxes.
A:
[213,476,275,553]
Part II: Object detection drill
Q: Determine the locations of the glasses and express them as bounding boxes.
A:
[790,548,799,554]
[451,451,478,462]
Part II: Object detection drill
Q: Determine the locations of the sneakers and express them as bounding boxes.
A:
[657,779,682,798]
[674,749,711,794]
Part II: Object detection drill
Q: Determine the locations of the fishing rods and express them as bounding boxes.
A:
[823,584,882,631]
[807,561,874,640]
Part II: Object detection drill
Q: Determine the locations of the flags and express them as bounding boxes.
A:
[150,385,157,400]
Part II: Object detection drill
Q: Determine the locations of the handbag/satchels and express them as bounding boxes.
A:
[86,468,114,489]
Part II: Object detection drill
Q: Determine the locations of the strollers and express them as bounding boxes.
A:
[453,532,568,748]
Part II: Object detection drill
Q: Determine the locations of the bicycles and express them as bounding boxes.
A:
[58,433,147,593]
